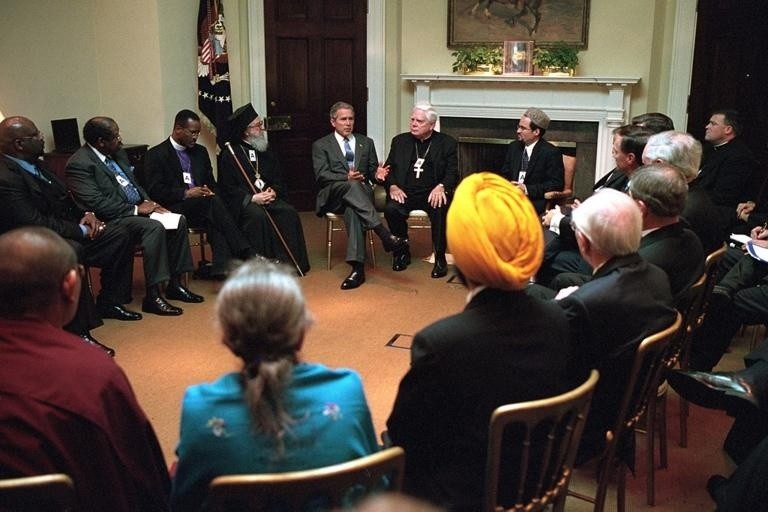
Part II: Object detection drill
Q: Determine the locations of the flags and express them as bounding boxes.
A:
[197,2,232,156]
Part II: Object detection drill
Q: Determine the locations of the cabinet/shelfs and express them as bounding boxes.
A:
[38,141,150,208]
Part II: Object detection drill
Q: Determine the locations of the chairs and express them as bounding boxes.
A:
[741,322,761,356]
[541,152,578,219]
[564,307,683,510]
[198,443,409,512]
[1,466,83,510]
[650,241,729,468]
[482,366,603,509]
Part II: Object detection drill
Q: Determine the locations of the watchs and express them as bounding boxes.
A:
[437,181,445,188]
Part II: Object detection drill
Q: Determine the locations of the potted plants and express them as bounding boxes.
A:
[445,42,504,79]
[529,38,580,77]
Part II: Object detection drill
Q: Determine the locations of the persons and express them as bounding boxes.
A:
[0,115,143,358]
[64,117,204,315]
[501,106,564,214]
[145,110,279,278]
[385,172,600,510]
[217,102,310,279]
[312,102,409,289]
[384,102,458,278]
[171,257,380,508]
[1,226,172,510]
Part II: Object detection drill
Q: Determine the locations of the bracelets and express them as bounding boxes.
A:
[84,211,95,216]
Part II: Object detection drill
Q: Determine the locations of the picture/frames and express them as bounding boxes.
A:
[444,0,590,52]
[501,38,536,77]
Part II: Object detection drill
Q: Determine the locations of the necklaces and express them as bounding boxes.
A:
[239,144,266,191]
[413,137,432,178]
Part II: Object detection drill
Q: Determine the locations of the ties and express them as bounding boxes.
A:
[343,136,355,171]
[103,158,138,204]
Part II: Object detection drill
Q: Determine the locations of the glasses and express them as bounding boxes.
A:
[16,132,45,142]
[182,128,201,136]
[99,135,122,142]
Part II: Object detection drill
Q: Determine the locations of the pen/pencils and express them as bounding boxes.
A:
[759,222,767,235]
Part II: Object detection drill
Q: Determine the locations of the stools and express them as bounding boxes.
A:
[84,222,210,308]
[322,210,386,274]
[387,207,436,265]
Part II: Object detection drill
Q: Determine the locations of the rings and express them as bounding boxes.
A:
[98,227,105,230]
[154,202,157,206]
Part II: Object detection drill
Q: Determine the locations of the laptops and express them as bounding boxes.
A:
[51,117,81,154]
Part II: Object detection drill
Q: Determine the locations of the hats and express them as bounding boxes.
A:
[622,113,675,135]
[225,104,260,130]
[523,107,551,130]
[444,169,547,294]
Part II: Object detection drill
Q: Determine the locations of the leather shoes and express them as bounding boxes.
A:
[167,284,204,303]
[78,332,114,357]
[341,271,365,289]
[393,252,410,270]
[665,368,761,420]
[432,259,448,277]
[143,297,182,316]
[97,304,143,320]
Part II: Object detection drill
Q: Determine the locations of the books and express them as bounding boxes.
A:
[149,209,186,231]
